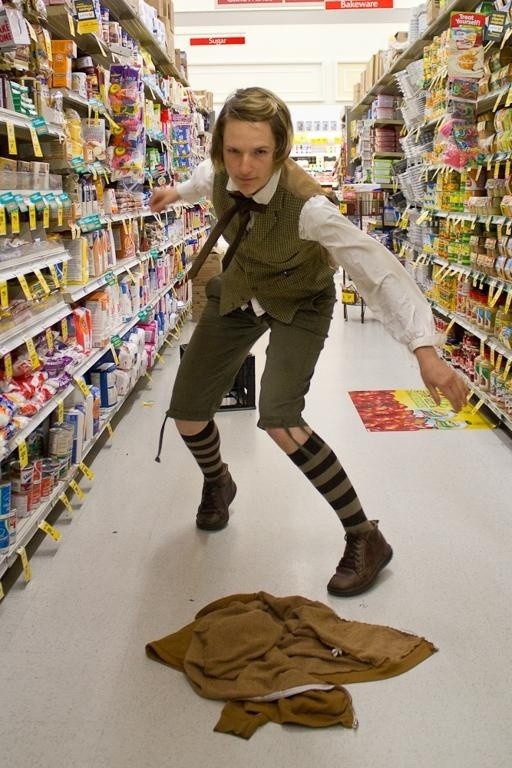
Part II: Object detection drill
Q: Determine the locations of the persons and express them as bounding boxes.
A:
[147,80,471,599]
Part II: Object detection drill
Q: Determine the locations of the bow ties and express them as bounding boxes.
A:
[187,190,266,281]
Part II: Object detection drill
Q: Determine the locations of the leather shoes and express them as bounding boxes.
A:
[327,519,393,597]
[196,470,237,533]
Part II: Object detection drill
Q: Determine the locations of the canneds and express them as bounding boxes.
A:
[0,422,75,554]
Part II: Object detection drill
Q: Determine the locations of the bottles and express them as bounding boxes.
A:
[494,305,504,338]
[473,350,505,397]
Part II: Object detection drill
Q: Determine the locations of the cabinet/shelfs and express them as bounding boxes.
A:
[339,0,512,437]
[0,0,217,595]
